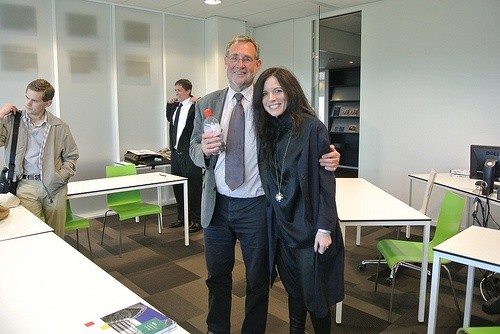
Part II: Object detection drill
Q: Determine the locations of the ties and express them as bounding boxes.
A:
[174,103,183,147]
[225,93,245,191]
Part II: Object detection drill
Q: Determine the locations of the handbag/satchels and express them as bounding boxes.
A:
[0,167,19,195]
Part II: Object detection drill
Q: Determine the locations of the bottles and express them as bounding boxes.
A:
[204,109,226,155]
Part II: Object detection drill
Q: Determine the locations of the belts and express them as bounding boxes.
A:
[173,149,184,155]
[22,174,41,181]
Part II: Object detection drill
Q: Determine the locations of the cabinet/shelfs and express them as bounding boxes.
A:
[328,67,360,169]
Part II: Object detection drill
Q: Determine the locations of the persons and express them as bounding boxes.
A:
[0,79,79,241]
[165,79,203,232]
[188,35,340,334]
[250,67,345,334]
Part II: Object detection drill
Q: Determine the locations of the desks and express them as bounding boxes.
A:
[67,172,190,246]
[114,161,172,234]
[406,174,500,238]
[335,178,432,324]
[0,204,55,242]
[0,232,191,334]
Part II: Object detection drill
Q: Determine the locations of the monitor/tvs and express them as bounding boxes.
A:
[470,145,500,196]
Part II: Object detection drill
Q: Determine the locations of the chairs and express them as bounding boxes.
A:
[427,225,500,334]
[101,164,164,258]
[66,198,92,261]
[374,192,464,325]
[358,170,437,286]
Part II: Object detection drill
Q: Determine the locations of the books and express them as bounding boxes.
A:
[83,302,178,334]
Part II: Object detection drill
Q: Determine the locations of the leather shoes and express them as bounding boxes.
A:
[189,221,203,233]
[168,219,192,227]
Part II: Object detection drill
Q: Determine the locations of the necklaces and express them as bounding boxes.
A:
[274,136,291,202]
